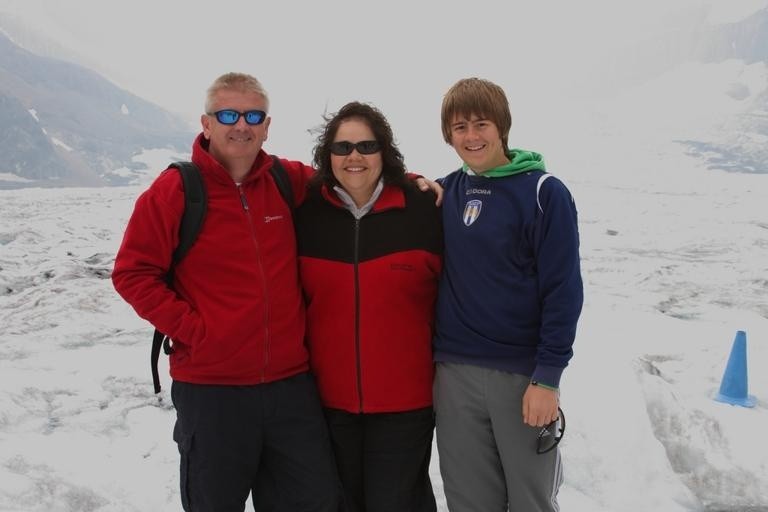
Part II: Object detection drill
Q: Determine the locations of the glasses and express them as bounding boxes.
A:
[206,108,266,126]
[328,140,383,156]
[536,406,565,454]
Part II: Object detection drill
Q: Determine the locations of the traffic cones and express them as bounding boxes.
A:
[720,331,749,400]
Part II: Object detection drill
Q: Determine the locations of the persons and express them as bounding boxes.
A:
[111,70,444,512]
[295,100,444,512]
[434,77,583,512]
